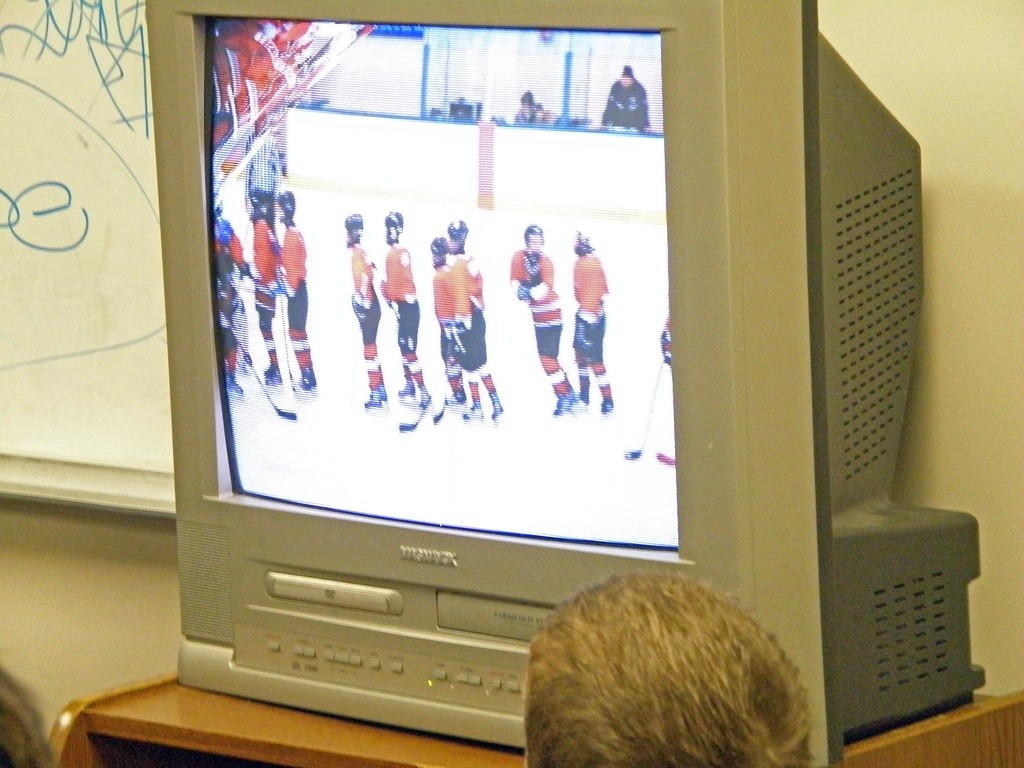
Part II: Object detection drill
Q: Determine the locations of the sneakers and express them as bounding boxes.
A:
[225,360,614,424]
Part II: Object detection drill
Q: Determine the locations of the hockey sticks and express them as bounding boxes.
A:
[371,261,399,321]
[622,361,675,464]
[280,296,298,393]
[232,327,297,421]
[398,372,448,432]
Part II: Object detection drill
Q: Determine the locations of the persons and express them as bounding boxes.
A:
[601,66,650,133]
[431,221,504,423]
[510,225,614,416]
[246,118,287,238]
[661,320,670,367]
[381,212,432,413]
[344,212,388,412]
[515,92,545,123]
[214,192,316,398]
[523,573,811,768]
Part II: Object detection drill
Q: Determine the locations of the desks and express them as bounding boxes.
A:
[43,674,1024,768]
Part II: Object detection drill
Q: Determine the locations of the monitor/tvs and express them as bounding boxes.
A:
[148,1,985,768]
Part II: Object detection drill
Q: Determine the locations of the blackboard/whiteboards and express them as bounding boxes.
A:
[0,0,174,522]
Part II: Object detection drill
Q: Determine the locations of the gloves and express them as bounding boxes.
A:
[661,330,671,366]
[574,322,594,351]
[517,284,533,302]
[351,290,364,320]
[269,231,282,255]
[240,263,256,281]
[444,322,468,342]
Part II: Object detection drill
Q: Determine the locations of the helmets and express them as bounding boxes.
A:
[574,230,596,258]
[431,237,450,268]
[248,189,270,223]
[345,214,363,248]
[447,220,468,256]
[385,212,404,245]
[276,192,296,223]
[212,192,222,218]
[525,225,543,254]
